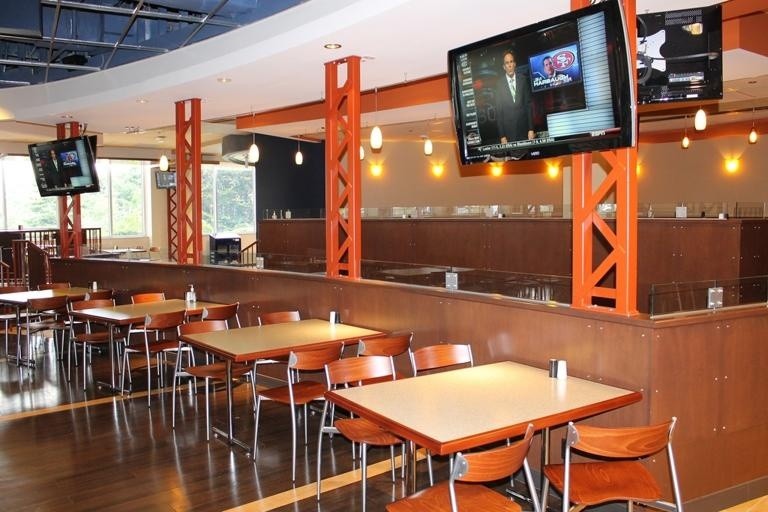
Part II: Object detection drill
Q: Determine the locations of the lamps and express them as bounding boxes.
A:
[422,137,434,157]
[368,122,383,151]
[247,142,262,166]
[747,125,758,143]
[159,151,172,175]
[692,108,708,133]
[295,149,306,166]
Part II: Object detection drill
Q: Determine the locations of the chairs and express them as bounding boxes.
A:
[252,343,344,483]
[61,290,116,368]
[36,283,71,362]
[0,286,30,359]
[315,356,406,512]
[120,310,188,409]
[356,330,415,444]
[401,343,474,488]
[178,303,241,386]
[171,321,259,443]
[16,294,69,370]
[251,310,315,417]
[128,292,167,344]
[541,415,683,512]
[385,424,541,512]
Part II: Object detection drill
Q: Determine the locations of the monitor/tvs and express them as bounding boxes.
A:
[89,135,97,163]
[448,0,636,165]
[156,171,176,189]
[28,135,100,197]
[636,4,724,104]
